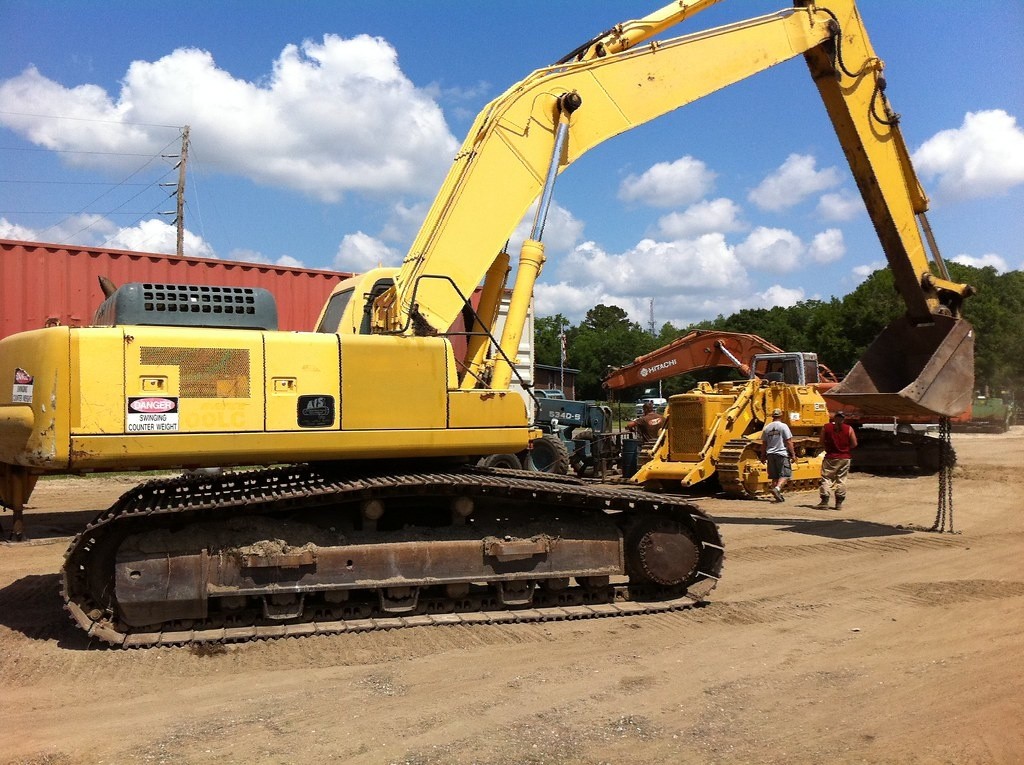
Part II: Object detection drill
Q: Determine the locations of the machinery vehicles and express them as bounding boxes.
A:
[0,2,988,653]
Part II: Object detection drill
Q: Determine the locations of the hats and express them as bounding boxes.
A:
[834,411,847,418]
[772,408,783,417]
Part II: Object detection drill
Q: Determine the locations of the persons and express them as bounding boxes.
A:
[817,411,858,510]
[760,409,798,502]
[627,402,668,449]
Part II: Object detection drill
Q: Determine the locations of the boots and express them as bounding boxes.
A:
[813,495,830,510]
[834,494,846,509]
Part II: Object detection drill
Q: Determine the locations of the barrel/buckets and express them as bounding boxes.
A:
[622,439,638,480]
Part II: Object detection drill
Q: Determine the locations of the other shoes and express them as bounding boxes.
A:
[770,488,784,502]
